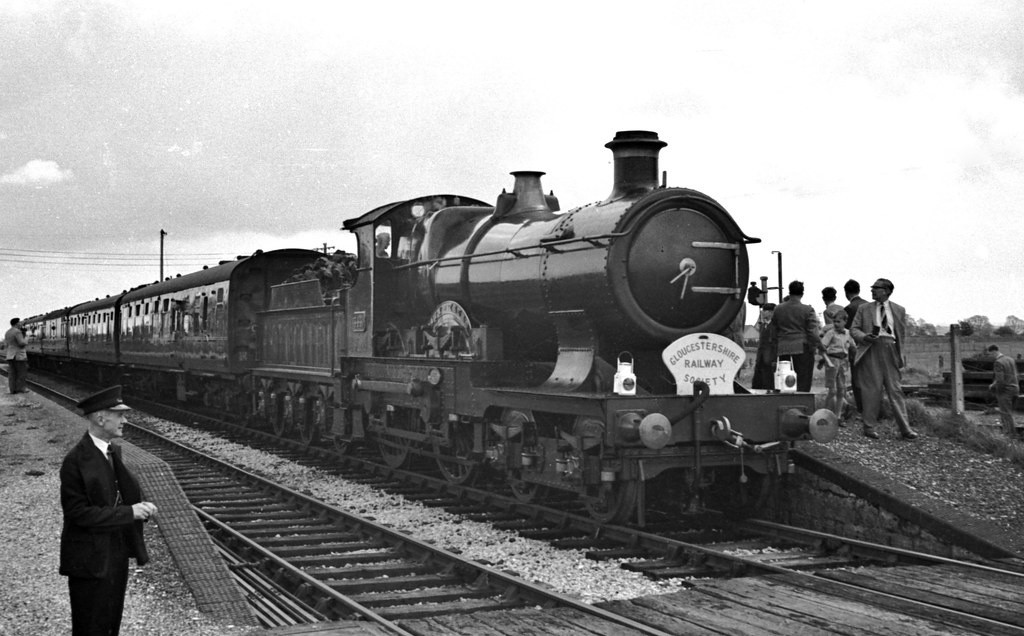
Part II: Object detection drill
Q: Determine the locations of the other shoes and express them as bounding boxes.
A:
[863,432,880,439]
[838,420,847,427]
[903,432,918,439]
[10,389,29,394]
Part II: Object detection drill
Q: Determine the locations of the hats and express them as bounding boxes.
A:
[77,385,132,415]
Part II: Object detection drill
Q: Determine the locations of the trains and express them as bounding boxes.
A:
[19,131,839,528]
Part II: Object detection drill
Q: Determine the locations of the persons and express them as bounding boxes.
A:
[987,344,1024,438]
[375,233,390,257]
[59,385,158,636]
[172,300,182,316]
[5,318,30,394]
[721,278,918,439]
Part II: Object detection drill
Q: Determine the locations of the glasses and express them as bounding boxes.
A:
[871,286,886,289]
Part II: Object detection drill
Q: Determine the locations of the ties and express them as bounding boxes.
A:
[108,445,114,469]
[880,302,892,334]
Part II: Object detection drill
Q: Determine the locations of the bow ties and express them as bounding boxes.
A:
[838,330,845,334]
